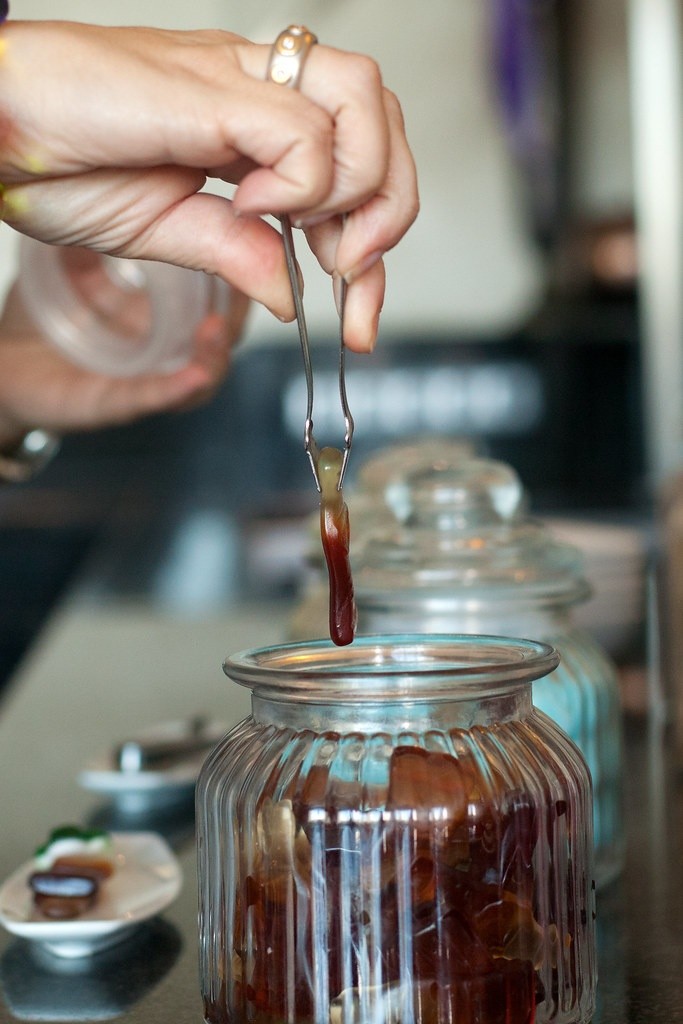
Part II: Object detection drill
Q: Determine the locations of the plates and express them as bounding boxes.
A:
[76,716,236,816]
[0,830,184,959]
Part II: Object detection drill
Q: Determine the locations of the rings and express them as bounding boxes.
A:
[266,25,317,91]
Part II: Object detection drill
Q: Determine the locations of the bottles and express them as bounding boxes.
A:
[299,459,628,1024]
[194,634,598,1024]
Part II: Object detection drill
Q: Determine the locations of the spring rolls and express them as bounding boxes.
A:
[40,859,112,918]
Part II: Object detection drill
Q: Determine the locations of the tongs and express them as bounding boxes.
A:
[114,717,223,772]
[278,205,356,490]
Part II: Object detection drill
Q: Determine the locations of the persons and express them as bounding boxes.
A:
[471,218,653,525]
[0,0,420,446]
